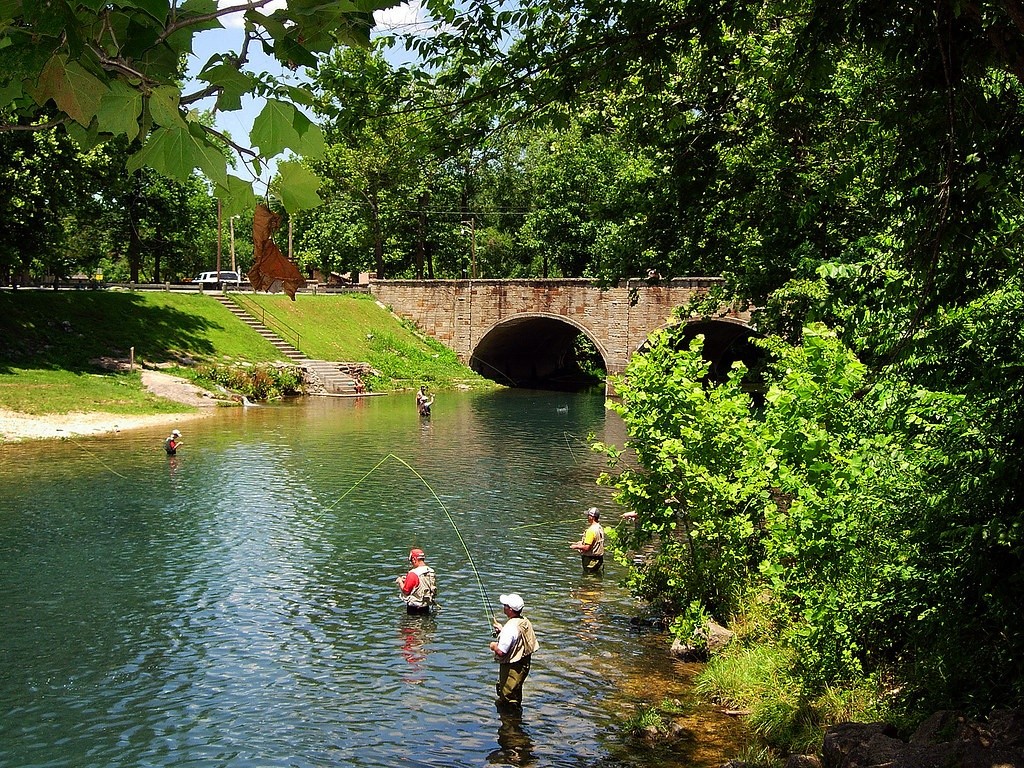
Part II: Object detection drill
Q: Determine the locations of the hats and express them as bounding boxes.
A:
[408,549,425,560]
[421,396,428,400]
[584,507,599,517]
[172,430,182,438]
[499,594,524,612]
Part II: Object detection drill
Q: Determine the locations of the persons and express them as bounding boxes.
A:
[354,374,366,394]
[568,507,605,576]
[165,429,183,455]
[490,593,541,715]
[419,393,434,416]
[417,384,426,413]
[396,548,437,615]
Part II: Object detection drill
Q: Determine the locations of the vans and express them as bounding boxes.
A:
[191,271,241,283]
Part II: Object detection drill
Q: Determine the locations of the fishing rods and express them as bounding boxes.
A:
[508,517,635,531]
[307,454,501,640]
[563,431,594,463]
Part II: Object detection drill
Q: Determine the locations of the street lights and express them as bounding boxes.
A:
[230,215,240,271]
[460,218,476,278]
